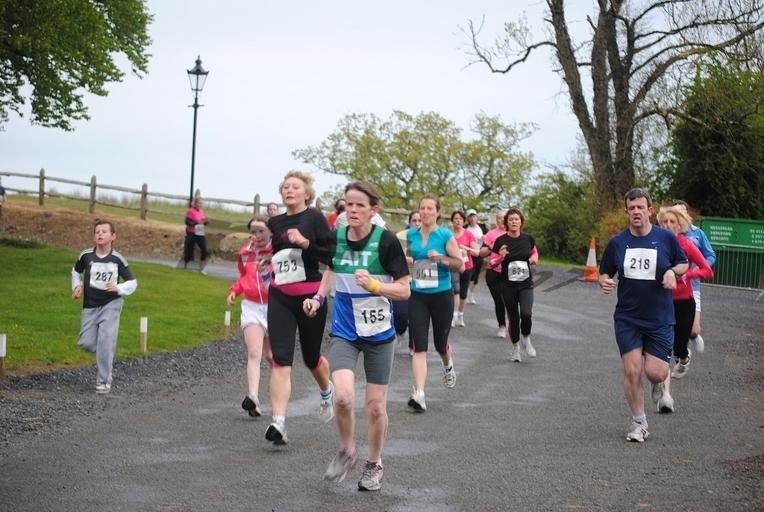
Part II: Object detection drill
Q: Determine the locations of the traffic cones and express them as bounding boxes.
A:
[581,235,606,282]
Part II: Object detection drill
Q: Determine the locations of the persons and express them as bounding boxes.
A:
[267,169,334,445]
[227,218,273,417]
[267,202,278,218]
[449,211,480,328]
[405,210,421,356]
[185,196,211,275]
[491,208,538,362]
[72,221,138,393]
[480,210,509,338]
[302,182,411,490]
[673,200,717,354]
[328,199,346,231]
[598,190,689,443]
[656,206,714,380]
[463,209,491,304]
[405,196,465,411]
[332,211,388,231]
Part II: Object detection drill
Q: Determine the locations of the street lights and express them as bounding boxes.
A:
[185,55,211,209]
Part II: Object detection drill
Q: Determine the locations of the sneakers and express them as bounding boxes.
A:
[458,319,465,327]
[470,297,476,304]
[511,345,522,363]
[658,392,674,413]
[497,328,508,338]
[466,298,470,304]
[690,335,705,354]
[322,446,359,484]
[240,394,262,416]
[318,380,335,423]
[440,357,457,389]
[451,319,456,327]
[524,338,536,357]
[357,459,384,491]
[407,385,427,412]
[95,383,111,394]
[670,347,692,380]
[650,383,666,404]
[626,417,649,442]
[264,423,290,446]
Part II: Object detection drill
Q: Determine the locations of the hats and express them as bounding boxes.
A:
[465,209,477,217]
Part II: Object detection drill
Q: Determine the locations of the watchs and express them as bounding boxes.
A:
[669,267,676,276]
